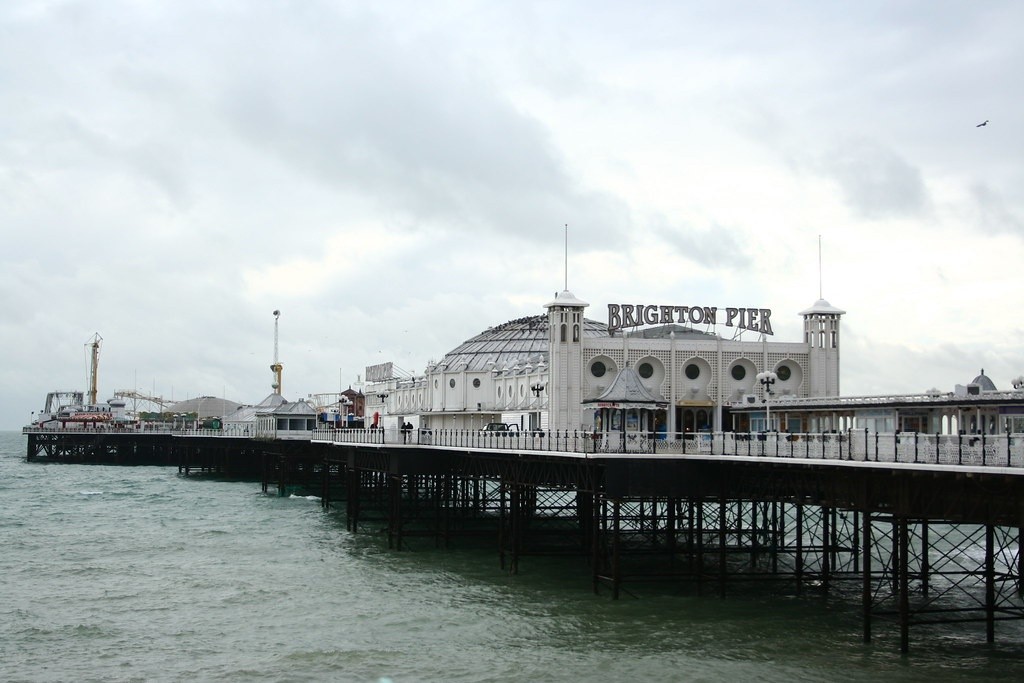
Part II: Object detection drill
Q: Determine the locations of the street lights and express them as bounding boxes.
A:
[529,378,546,431]
[376,388,391,428]
[756,371,777,432]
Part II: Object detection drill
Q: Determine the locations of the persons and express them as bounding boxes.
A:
[401,422,406,444]
[406,422,414,444]
